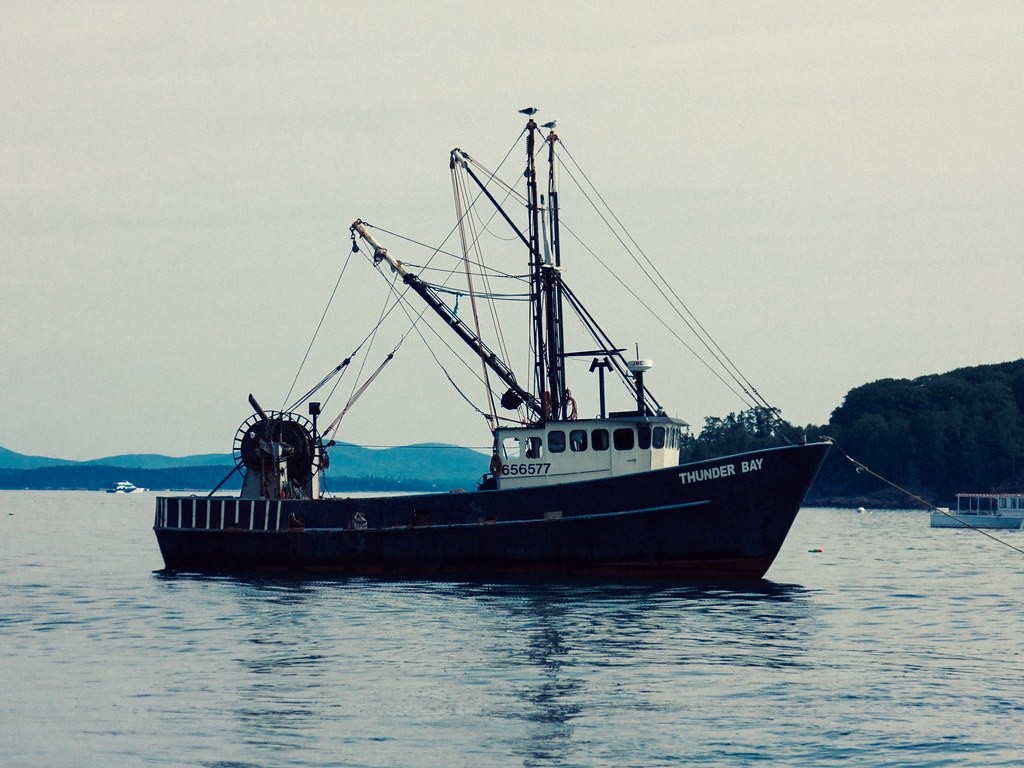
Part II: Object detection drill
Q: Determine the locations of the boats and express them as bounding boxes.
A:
[929,492,1024,529]
[106,480,150,493]
[152,104,838,585]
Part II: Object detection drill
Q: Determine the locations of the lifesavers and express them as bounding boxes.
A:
[489,455,501,475]
